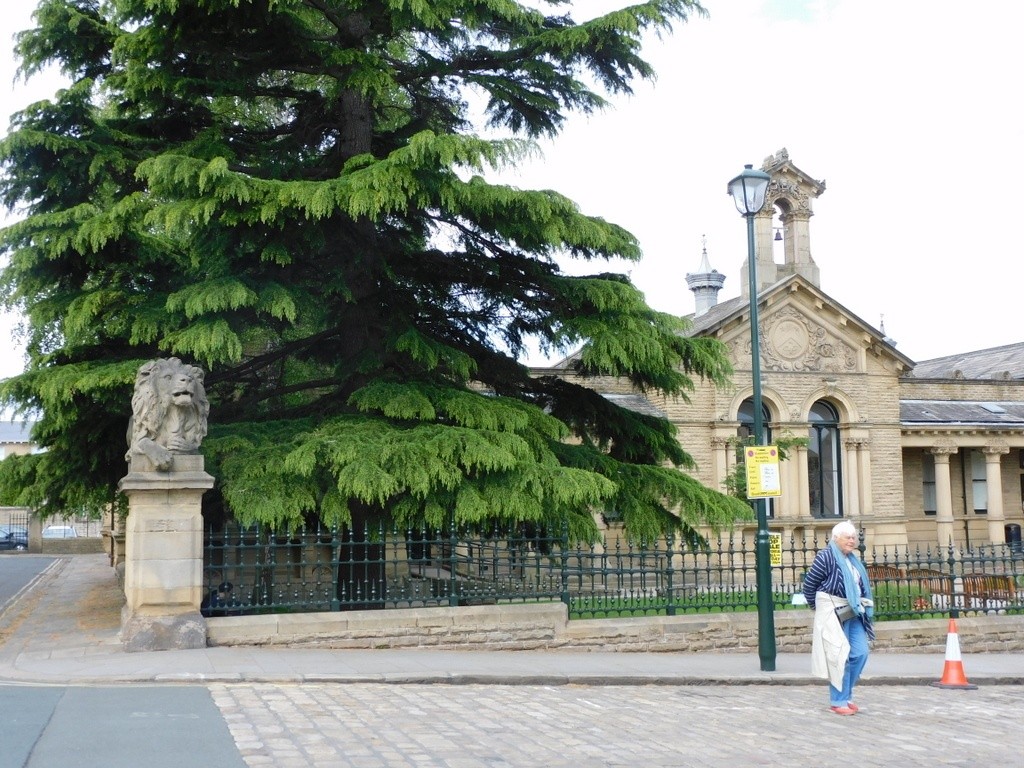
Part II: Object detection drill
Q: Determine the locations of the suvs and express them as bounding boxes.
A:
[42,525,81,538]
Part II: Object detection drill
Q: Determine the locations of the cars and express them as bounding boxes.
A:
[0,526,28,551]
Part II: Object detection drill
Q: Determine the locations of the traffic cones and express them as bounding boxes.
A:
[928,618,978,690]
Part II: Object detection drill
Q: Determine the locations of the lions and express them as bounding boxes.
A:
[125,358,211,470]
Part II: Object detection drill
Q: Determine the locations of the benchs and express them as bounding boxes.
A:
[863,565,1015,606]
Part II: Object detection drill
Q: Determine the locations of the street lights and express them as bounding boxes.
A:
[727,162,776,674]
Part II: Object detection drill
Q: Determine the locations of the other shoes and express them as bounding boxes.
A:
[848,701,858,711]
[831,706,854,716]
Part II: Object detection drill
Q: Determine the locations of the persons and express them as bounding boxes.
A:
[802,521,874,716]
